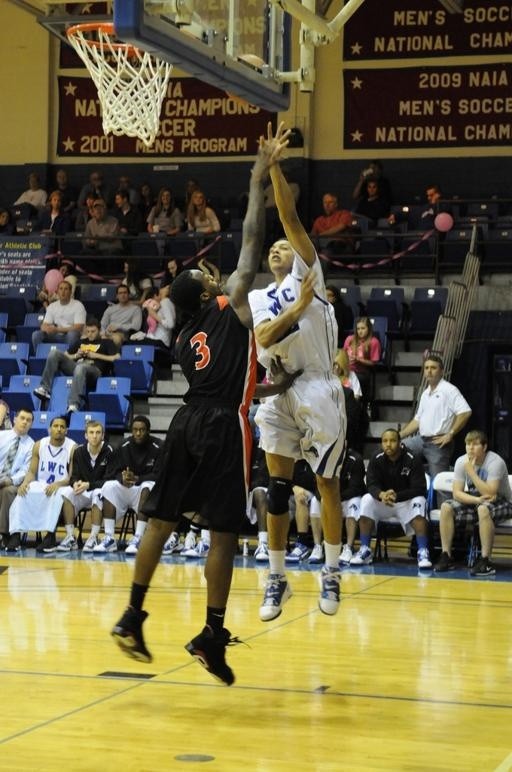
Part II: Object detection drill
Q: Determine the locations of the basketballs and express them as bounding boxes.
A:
[224,55,266,105]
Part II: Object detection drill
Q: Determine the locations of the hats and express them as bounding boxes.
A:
[93,199,106,208]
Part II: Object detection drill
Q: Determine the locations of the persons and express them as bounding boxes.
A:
[0,123,508,556]
[113,122,351,684]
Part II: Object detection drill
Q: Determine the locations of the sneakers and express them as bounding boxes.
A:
[317,565,341,616]
[109,608,152,663]
[66,404,79,415]
[33,387,51,402]
[258,577,294,623]
[184,630,234,688]
[1,538,500,577]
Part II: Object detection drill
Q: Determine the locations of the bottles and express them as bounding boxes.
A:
[242,536,249,557]
